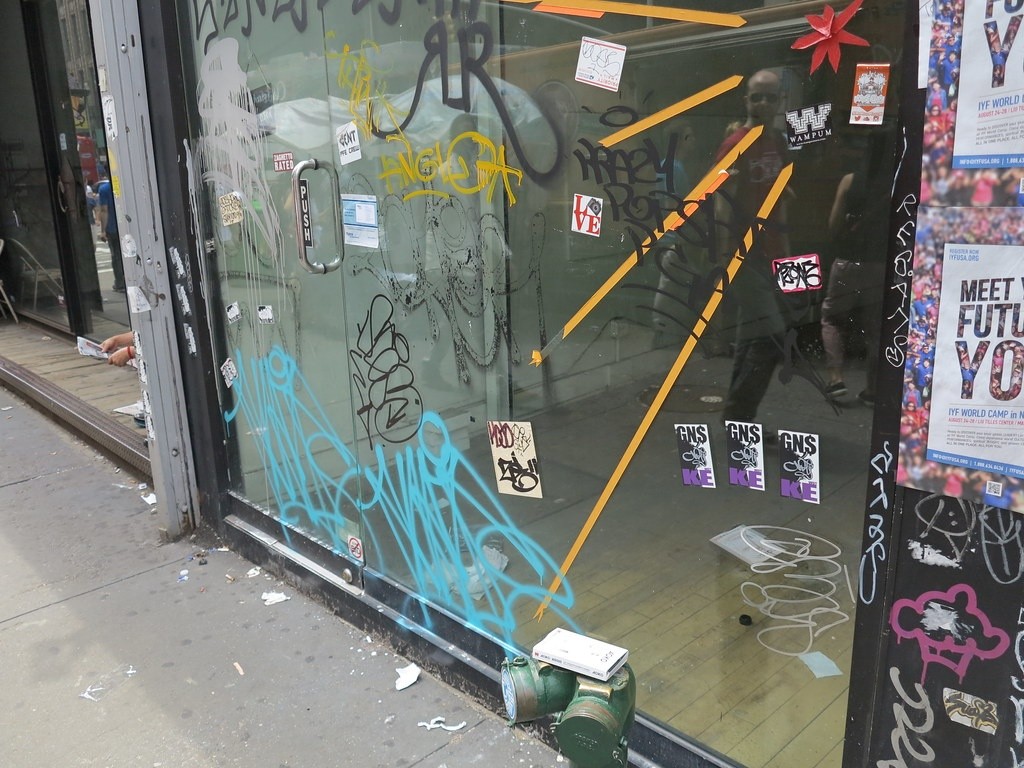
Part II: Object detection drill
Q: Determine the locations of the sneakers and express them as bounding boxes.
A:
[855,390,875,407]
[825,381,847,399]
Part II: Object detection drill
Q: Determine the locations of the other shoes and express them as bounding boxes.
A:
[721,418,776,443]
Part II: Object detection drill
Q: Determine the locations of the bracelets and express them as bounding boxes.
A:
[127,346,133,359]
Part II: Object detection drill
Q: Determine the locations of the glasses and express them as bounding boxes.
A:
[750,93,778,104]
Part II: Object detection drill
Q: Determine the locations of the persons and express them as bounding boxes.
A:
[100,331,148,447]
[425,114,523,400]
[895,0,1024,512]
[268,172,333,328]
[654,69,887,448]
[85,161,125,293]
